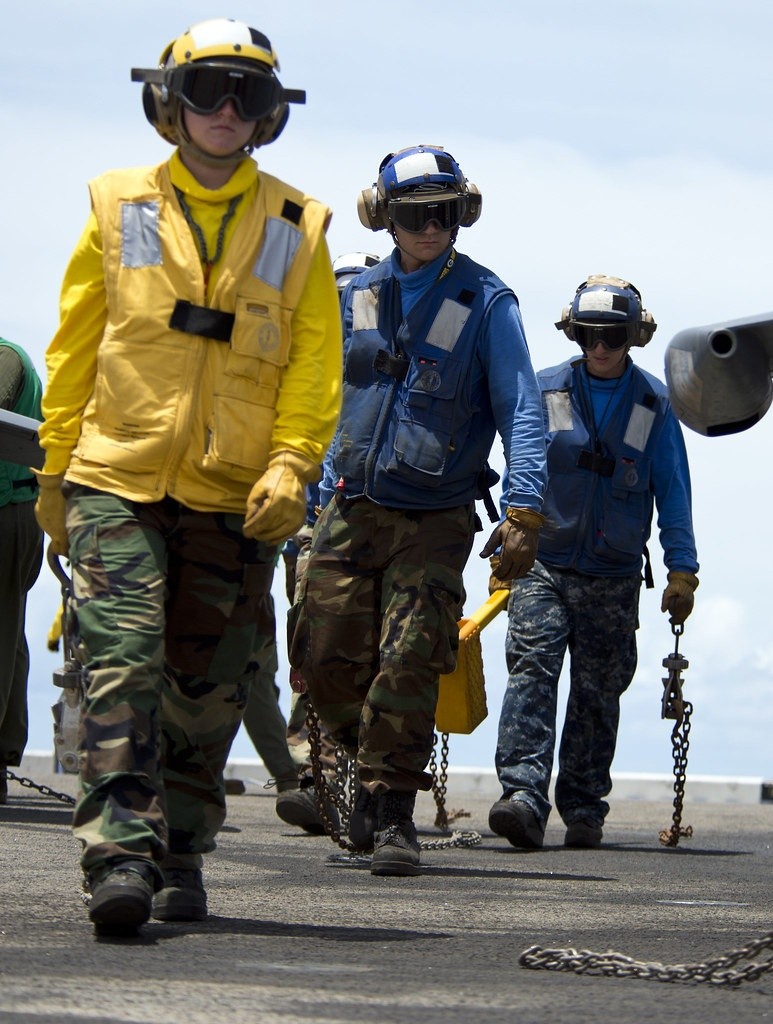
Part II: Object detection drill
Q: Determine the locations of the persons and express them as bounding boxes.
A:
[298,146,549,876]
[489,275,700,849]
[0,336,45,805]
[30,18,342,931]
[223,250,380,838]
[47,595,71,652]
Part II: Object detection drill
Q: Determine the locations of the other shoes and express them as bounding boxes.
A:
[263,778,297,792]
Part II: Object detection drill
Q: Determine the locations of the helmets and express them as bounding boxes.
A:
[568,275,643,358]
[164,21,280,143]
[375,146,467,235]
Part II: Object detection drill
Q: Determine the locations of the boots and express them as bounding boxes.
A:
[372,790,420,874]
[349,757,377,849]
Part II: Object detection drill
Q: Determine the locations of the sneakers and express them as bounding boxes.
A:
[277,786,341,835]
[90,870,152,932]
[151,869,207,920]
[565,818,602,847]
[489,799,543,850]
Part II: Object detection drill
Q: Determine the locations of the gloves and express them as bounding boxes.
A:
[241,449,322,546]
[479,506,546,580]
[28,466,69,560]
[661,571,699,625]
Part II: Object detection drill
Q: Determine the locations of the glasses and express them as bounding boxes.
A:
[170,63,283,120]
[572,322,632,352]
[384,191,467,233]
